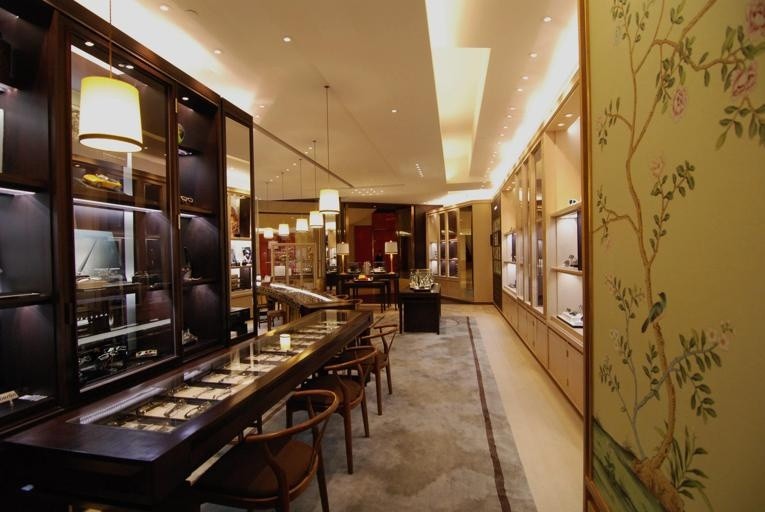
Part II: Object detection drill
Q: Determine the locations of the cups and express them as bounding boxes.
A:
[409,272,433,289]
[279,333,291,350]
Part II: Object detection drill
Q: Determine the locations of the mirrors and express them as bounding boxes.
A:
[220,95,258,350]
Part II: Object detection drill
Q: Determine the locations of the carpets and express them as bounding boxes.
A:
[203,292,542,511]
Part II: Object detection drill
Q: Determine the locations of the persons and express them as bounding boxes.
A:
[374,251,382,266]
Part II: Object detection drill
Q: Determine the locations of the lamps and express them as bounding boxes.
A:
[385,239,400,275]
[337,242,351,275]
[77,2,145,152]
[259,77,342,240]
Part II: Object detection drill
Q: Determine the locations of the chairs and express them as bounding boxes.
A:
[205,389,340,508]
[297,346,379,475]
[358,324,398,394]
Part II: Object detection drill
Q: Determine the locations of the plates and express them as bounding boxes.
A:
[354,277,374,282]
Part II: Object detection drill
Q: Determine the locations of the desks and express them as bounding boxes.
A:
[400,278,443,334]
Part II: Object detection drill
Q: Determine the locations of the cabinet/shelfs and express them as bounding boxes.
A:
[6,272,73,437]
[0,1,73,438]
[425,199,494,305]
[6,308,373,502]
[271,241,318,291]
[220,274,259,351]
[225,186,256,319]
[492,64,584,420]
[62,0,180,416]
[65,271,173,412]
[170,273,229,370]
[171,63,228,371]
